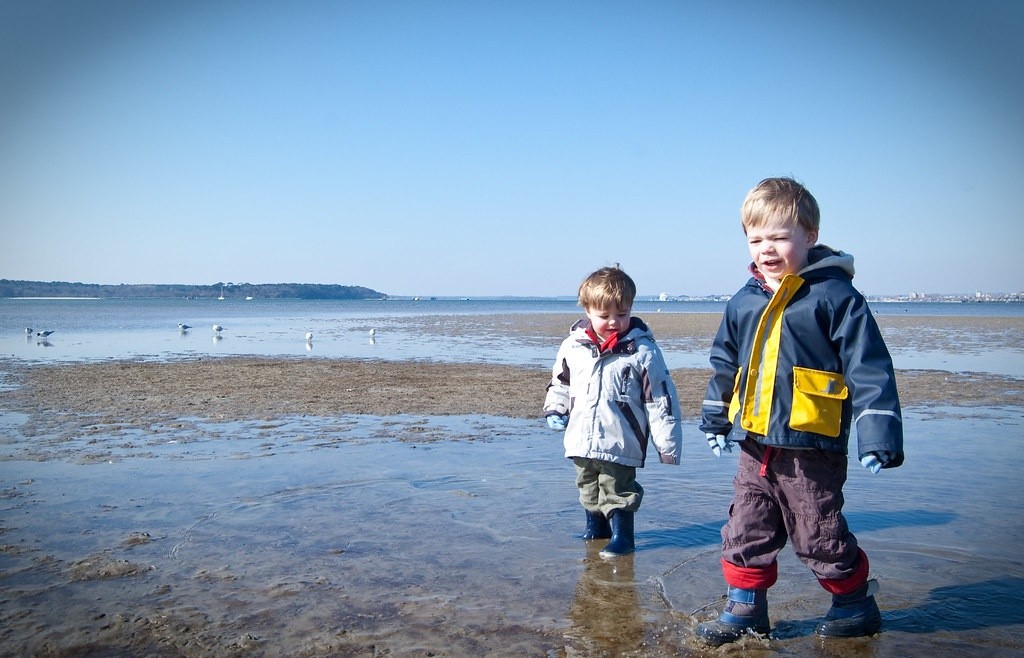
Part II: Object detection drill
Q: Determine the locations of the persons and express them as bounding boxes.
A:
[697,180,905,646]
[543,267,682,561]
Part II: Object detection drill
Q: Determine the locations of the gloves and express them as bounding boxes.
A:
[547,415,568,431]
[861,450,896,474]
[706,432,732,458]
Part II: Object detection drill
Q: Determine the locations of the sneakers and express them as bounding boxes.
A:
[695,584,771,646]
[814,578,881,637]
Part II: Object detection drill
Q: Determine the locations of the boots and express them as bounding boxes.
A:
[598,508,635,558]
[573,509,612,539]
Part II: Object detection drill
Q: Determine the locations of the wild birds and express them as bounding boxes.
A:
[37,330,55,339]
[178,323,192,332]
[25,327,34,336]
[874,306,908,314]
[211,324,226,335]
[306,332,313,341]
[644,308,662,325]
[369,328,377,335]
